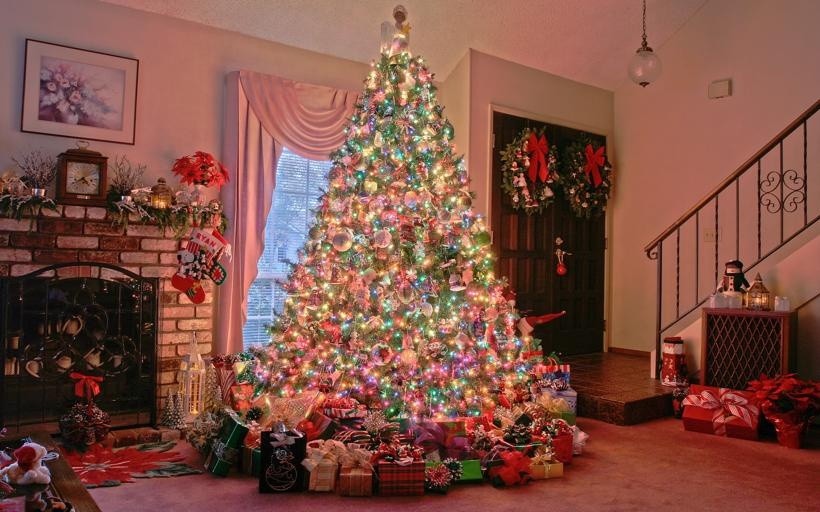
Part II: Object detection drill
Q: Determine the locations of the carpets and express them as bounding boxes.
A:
[56,435,203,489]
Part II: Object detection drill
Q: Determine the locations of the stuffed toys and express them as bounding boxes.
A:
[0,440,52,485]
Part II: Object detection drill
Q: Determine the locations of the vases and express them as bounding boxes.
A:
[191,185,206,207]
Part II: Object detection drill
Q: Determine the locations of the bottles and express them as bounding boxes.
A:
[774,295,791,311]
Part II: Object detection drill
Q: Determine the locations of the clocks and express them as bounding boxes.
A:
[55,141,109,206]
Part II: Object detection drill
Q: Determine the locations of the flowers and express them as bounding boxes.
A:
[171,150,232,192]
[499,125,566,218]
[562,134,612,219]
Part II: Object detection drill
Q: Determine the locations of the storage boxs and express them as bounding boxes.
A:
[200,358,578,498]
[682,383,761,441]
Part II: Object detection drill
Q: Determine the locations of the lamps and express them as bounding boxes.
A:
[151,177,173,208]
[627,4,663,89]
[745,272,771,311]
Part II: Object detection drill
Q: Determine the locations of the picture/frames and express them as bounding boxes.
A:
[20,38,140,146]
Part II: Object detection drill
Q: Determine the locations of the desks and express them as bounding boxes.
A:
[0,430,103,512]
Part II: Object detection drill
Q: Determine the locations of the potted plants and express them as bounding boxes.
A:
[745,370,820,449]
[108,151,147,201]
[8,146,58,196]
